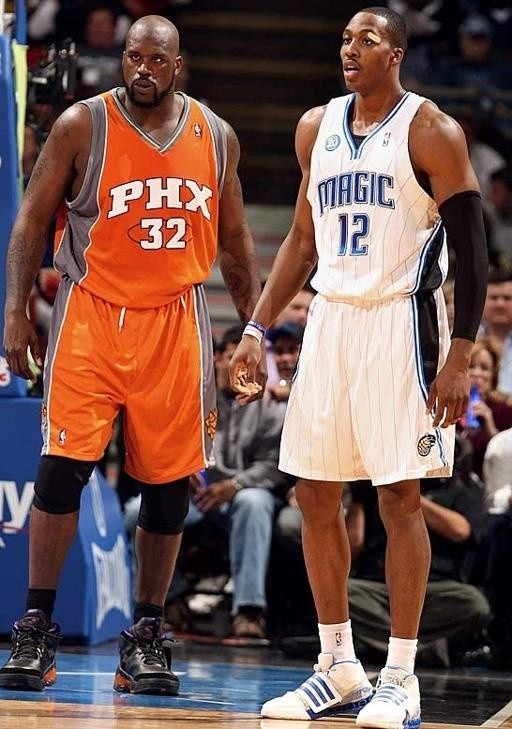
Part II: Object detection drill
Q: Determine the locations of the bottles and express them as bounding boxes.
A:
[464,383,481,429]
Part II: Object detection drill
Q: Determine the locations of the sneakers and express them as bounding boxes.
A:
[233,610,265,639]
[260,654,374,720]
[113,616,180,694]
[448,635,497,671]
[355,667,421,729]
[1,609,60,691]
